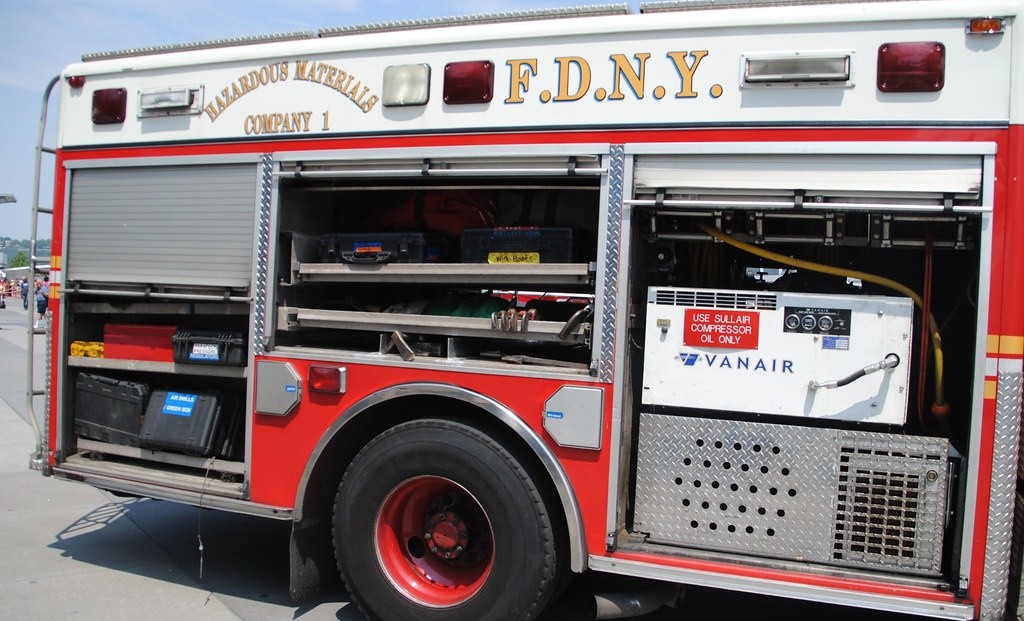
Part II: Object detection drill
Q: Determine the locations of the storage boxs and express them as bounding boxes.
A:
[172,330,248,367]
[315,232,425,263]
[75,371,245,463]
[460,225,573,264]
[103,322,178,362]
[70,340,103,358]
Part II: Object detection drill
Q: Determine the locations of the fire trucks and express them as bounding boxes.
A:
[22,2,1023,620]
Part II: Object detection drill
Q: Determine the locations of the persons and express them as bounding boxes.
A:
[0,272,49,328]
